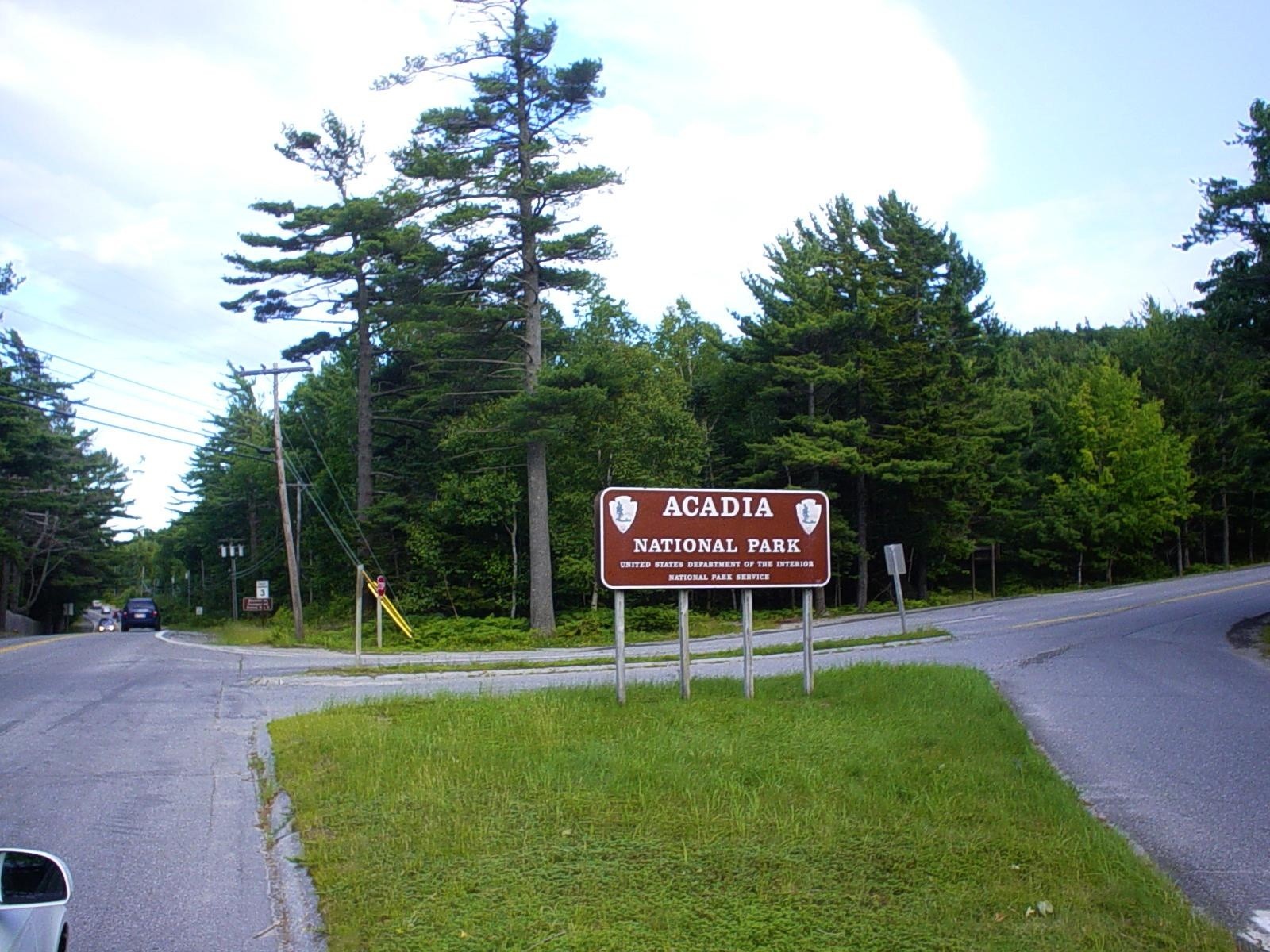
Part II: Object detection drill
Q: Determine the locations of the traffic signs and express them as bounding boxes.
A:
[599,486,831,588]
[243,597,273,611]
[256,580,269,598]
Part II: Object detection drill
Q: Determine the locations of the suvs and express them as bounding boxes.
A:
[121,597,161,632]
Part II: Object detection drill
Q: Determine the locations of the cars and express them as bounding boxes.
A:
[112,610,123,623]
[0,847,76,952]
[97,618,115,632]
[91,600,102,609]
[101,606,111,613]
